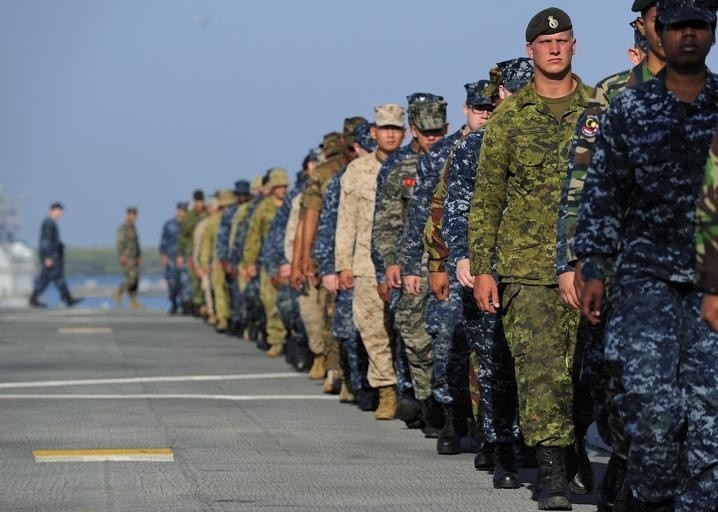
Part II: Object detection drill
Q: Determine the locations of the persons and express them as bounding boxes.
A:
[158,0,718,512]
[113,206,145,309]
[28,202,83,311]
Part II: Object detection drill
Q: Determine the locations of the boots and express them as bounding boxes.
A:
[234,324,398,420]
[397,397,627,512]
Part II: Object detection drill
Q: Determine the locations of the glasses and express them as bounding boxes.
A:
[472,106,493,115]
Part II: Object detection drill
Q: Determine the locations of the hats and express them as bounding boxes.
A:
[310,92,447,161]
[526,7,572,42]
[464,57,534,106]
[209,168,288,209]
[629,0,718,53]
